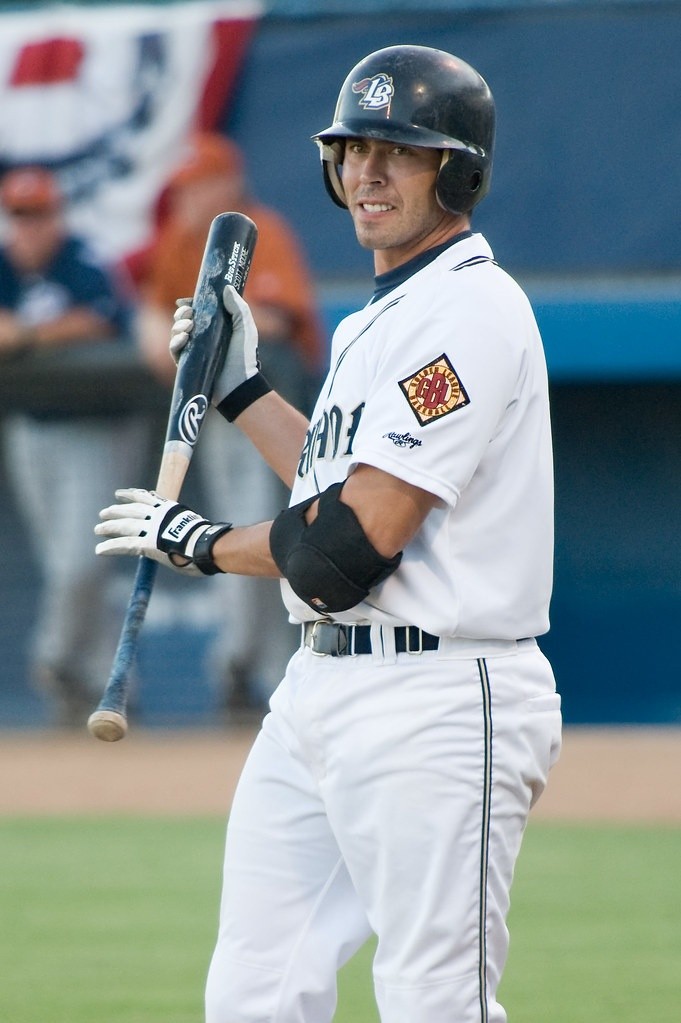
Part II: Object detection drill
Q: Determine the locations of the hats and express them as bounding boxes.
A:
[168,128,242,188]
[2,167,66,215]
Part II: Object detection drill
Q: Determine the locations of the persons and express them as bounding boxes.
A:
[135,130,323,708]
[94,44,562,1023]
[0,167,151,727]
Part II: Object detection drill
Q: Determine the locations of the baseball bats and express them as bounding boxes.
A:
[86,212,261,742]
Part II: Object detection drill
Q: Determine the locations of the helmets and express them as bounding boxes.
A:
[310,44,498,214]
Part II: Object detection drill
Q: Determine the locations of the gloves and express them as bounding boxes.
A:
[169,285,273,424]
[93,487,233,578]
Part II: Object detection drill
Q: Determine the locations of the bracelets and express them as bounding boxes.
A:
[193,521,232,574]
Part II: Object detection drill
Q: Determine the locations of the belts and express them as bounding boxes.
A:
[304,622,440,655]
